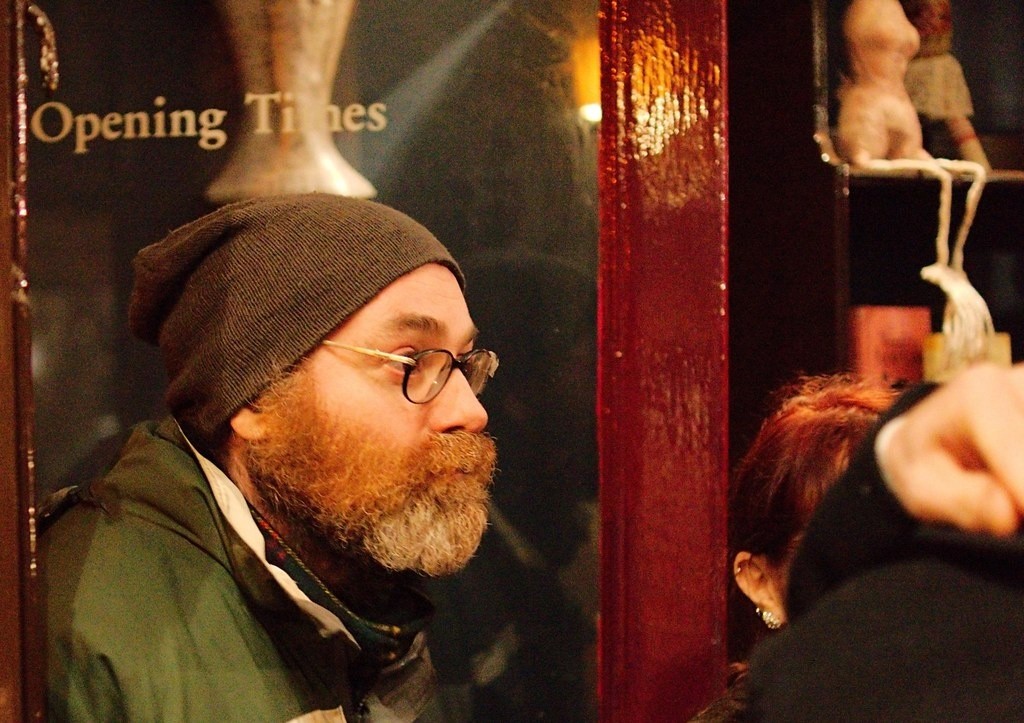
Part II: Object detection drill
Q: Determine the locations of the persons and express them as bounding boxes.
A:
[33,190,497,722]
[690,364,1024,723]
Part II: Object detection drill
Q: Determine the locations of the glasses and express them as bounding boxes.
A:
[319,339,493,405]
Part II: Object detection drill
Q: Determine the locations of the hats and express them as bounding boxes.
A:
[126,195,468,439]
